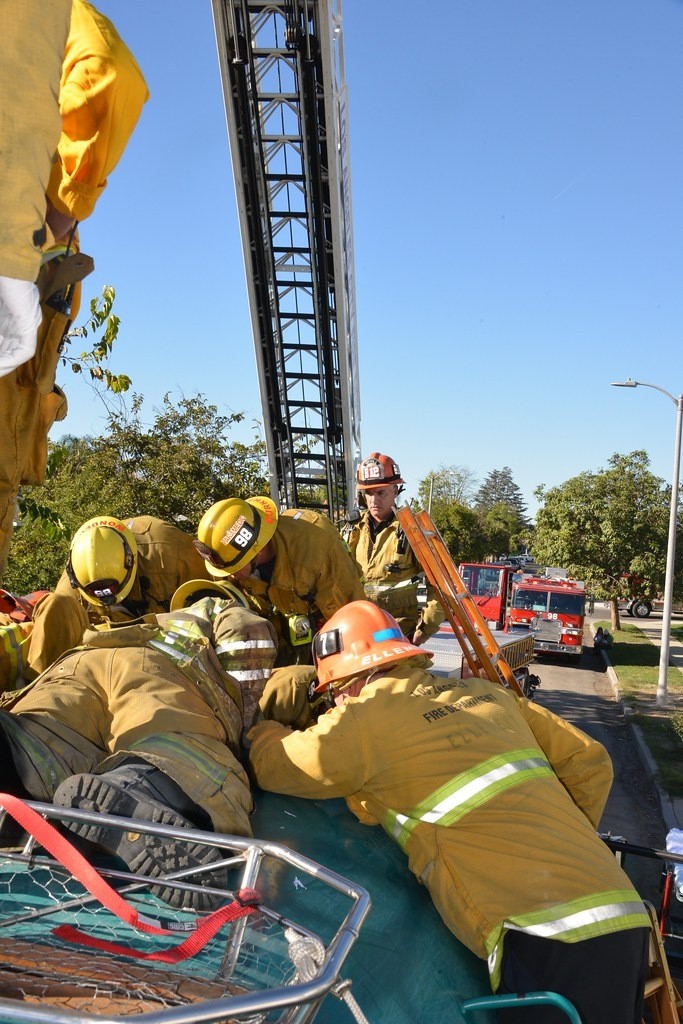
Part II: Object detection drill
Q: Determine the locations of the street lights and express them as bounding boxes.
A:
[428,472,461,518]
[611,378,683,708]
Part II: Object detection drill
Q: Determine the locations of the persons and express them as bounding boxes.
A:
[0,0,150,579]
[340,452,446,646]
[593,627,614,656]
[0,495,367,910]
[240,601,653,1024]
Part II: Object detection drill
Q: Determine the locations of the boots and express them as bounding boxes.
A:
[52,761,228,910]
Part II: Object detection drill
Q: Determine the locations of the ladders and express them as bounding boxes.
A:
[394,505,525,698]
[638,899,680,1024]
[497,635,535,677]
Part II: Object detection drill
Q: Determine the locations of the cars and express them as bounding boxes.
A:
[500,558,526,568]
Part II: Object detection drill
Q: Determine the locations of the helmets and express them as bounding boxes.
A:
[309,600,435,695]
[0,589,48,622]
[355,452,406,490]
[196,494,279,577]
[70,516,138,606]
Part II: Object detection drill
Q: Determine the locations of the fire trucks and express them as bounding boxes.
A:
[608,573,683,616]
[503,567,586,663]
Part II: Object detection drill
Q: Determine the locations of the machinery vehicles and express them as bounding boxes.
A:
[457,562,512,629]
